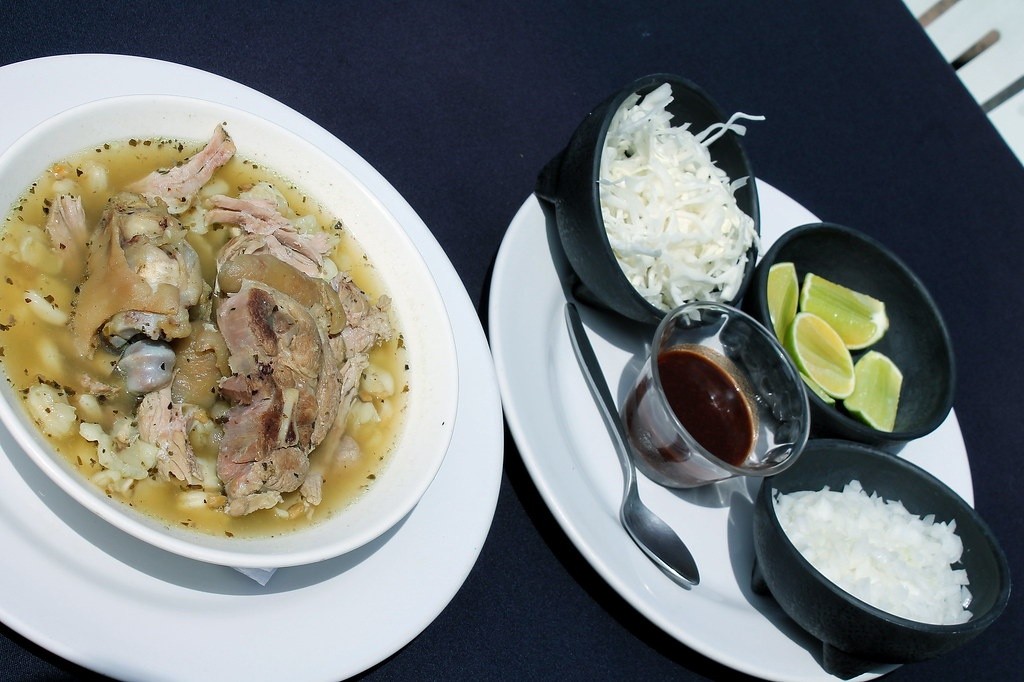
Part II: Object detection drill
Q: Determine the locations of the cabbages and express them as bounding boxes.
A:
[597,80,767,324]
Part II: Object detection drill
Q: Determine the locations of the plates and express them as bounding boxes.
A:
[487,176,973,682]
[0,93,459,568]
[0,54,501,682]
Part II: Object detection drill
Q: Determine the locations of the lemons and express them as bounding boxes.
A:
[766,261,904,432]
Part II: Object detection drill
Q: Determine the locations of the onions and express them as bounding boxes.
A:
[771,479,973,626]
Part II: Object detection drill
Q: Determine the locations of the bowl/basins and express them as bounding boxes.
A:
[535,72,760,330]
[750,438,1012,675]
[741,223,955,441]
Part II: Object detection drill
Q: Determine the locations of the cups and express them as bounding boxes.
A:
[620,301,810,489]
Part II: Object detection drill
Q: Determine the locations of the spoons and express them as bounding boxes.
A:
[566,303,700,586]
